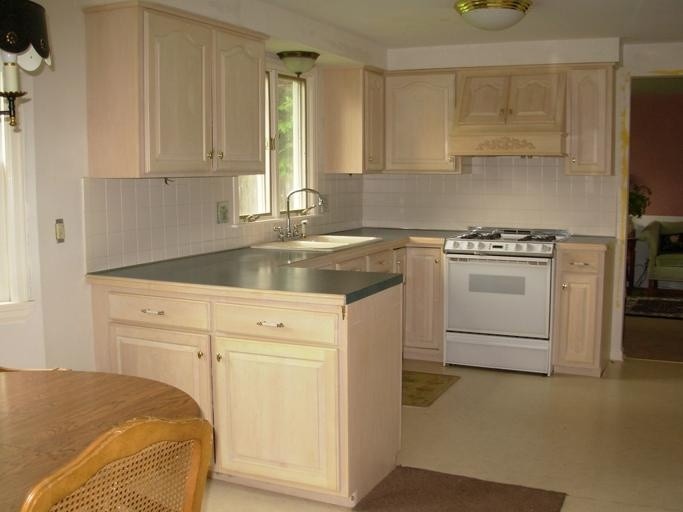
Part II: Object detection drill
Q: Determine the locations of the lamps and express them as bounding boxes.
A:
[453,1,534,30]
[0,0,54,130]
[276,52,320,78]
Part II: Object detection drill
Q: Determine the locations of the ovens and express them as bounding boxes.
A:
[441,253,556,378]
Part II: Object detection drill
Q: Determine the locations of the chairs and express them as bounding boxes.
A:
[640,220,683,290]
[23,417,215,512]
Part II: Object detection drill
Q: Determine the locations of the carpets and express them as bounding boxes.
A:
[624,295,683,319]
[350,465,566,512]
[402,370,460,408]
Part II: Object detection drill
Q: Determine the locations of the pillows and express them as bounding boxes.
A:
[658,233,683,253]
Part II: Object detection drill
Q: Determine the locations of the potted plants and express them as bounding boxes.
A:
[627,172,652,235]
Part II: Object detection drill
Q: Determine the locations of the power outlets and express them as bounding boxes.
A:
[217,201,229,224]
[55,223,65,240]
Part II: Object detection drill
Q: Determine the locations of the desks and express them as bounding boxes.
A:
[626,229,636,296]
[1,371,202,512]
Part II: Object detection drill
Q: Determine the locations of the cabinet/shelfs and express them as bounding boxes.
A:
[550,236,615,377]
[106,290,341,494]
[323,65,385,175]
[564,62,617,177]
[402,247,444,363]
[81,0,270,179]
[335,249,394,274]
[446,63,568,157]
[384,68,473,176]
[392,247,407,303]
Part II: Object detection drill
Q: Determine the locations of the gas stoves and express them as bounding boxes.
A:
[442,225,572,259]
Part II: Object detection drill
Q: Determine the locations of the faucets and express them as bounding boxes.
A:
[287,188,326,231]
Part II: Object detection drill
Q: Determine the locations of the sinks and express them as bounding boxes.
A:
[250,242,348,252]
[300,235,384,242]
